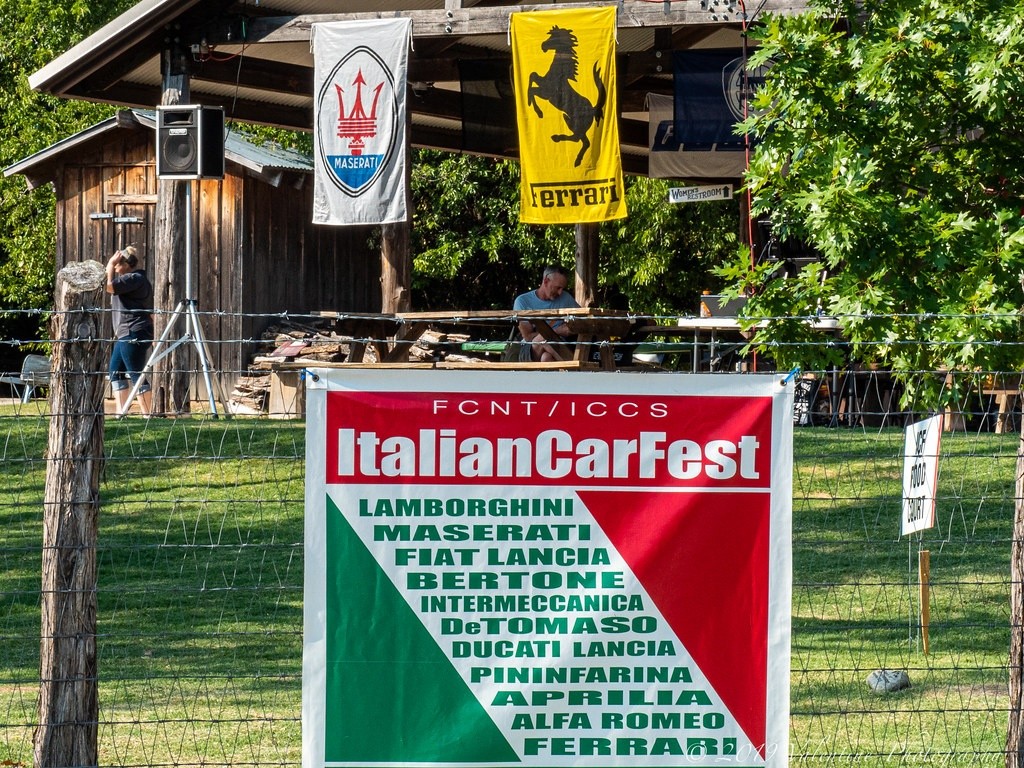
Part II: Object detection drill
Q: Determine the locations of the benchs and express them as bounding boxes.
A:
[258,361,597,420]
[0,353,52,403]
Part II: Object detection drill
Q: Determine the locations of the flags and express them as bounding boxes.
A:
[672,47,784,141]
[649,93,791,179]
[311,18,408,225]
[510,8,628,224]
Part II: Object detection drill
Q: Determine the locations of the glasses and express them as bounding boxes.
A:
[545,269,569,277]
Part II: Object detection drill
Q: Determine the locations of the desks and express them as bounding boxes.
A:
[313,307,655,370]
[643,326,847,421]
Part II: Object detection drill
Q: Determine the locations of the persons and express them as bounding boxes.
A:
[514,264,583,362]
[106,247,154,421]
